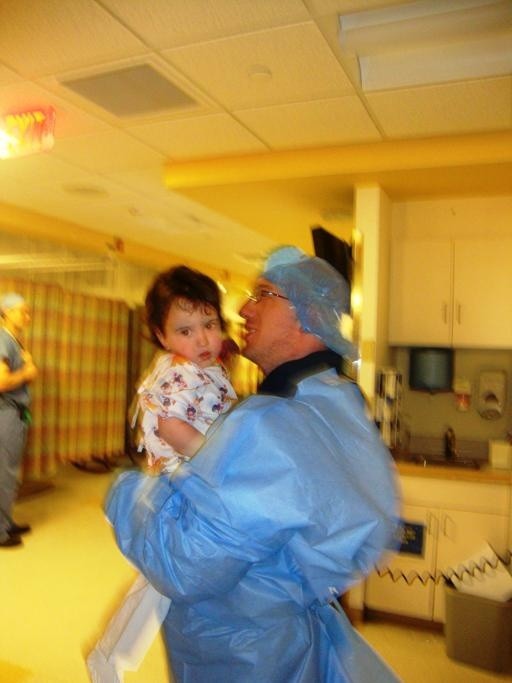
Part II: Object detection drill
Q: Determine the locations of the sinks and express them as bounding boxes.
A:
[411,453,479,470]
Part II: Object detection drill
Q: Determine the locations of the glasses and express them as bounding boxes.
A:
[249,288,289,300]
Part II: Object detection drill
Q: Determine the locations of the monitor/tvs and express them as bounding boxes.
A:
[312,227,351,313]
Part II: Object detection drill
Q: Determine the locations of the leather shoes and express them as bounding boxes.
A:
[1,522,31,548]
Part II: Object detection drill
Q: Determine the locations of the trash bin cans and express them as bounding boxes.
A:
[443,575,512,672]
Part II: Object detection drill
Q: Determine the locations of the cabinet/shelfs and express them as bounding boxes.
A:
[364,477,512,624]
[390,237,512,350]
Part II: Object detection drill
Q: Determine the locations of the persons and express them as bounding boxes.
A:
[101,245,400,681]
[0,295,38,546]
[88,264,242,681]
